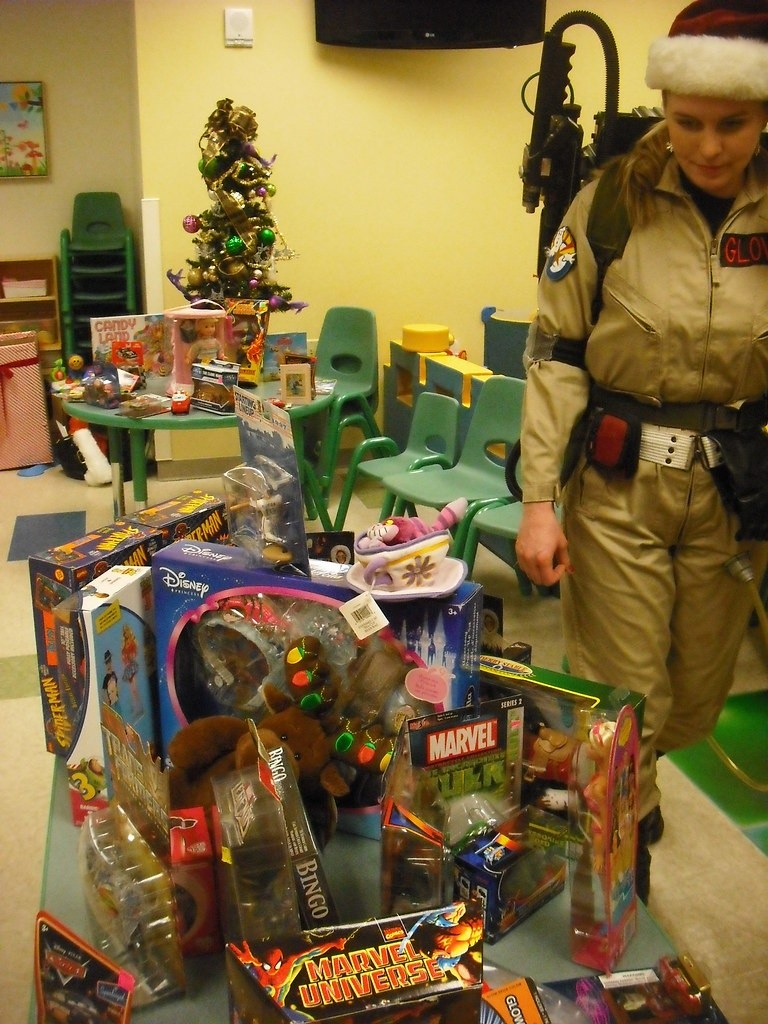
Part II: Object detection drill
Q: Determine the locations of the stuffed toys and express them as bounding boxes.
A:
[161,681,350,812]
[358,497,468,550]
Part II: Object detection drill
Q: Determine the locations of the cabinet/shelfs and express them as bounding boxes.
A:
[0,256,66,380]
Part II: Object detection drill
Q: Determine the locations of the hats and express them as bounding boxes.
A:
[646,1,768,103]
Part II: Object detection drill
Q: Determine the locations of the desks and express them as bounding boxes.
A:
[61,376,339,527]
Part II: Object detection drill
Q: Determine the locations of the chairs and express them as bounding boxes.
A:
[458,437,565,587]
[378,375,529,518]
[59,192,143,367]
[322,357,387,512]
[311,306,379,490]
[355,391,461,523]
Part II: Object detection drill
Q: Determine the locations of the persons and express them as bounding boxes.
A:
[184,317,229,367]
[121,623,145,719]
[100,650,121,718]
[582,720,619,936]
[514,0,768,908]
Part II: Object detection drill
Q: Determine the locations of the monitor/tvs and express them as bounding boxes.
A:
[313,0,548,50]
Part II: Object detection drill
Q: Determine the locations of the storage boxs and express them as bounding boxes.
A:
[0,334,55,473]
[259,331,308,383]
[0,273,48,300]
[0,318,56,345]
[187,358,242,416]
[21,489,734,1022]
[89,313,173,380]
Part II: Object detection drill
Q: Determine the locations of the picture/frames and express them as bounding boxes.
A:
[278,363,313,406]
[0,80,49,180]
[283,353,317,398]
